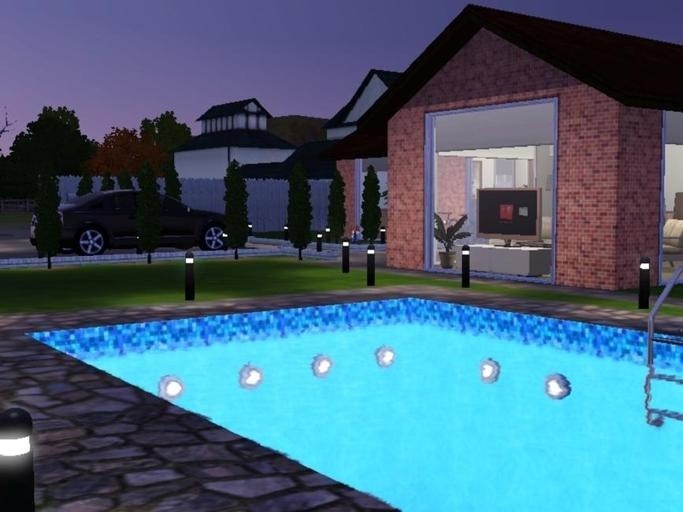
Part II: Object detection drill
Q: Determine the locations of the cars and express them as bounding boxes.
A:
[28,188,227,255]
